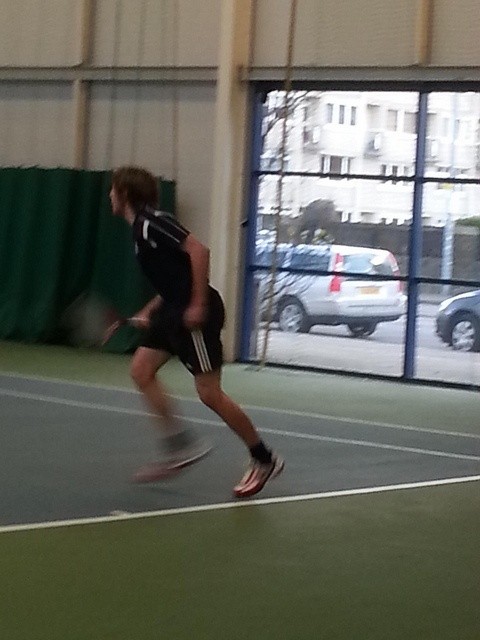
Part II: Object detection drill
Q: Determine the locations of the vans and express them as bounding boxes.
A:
[256,243,407,335]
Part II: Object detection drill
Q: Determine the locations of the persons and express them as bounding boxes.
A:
[108,166,286,498]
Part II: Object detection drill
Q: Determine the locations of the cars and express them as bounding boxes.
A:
[436,289,479,351]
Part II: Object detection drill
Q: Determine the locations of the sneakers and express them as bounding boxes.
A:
[232,449,285,498]
